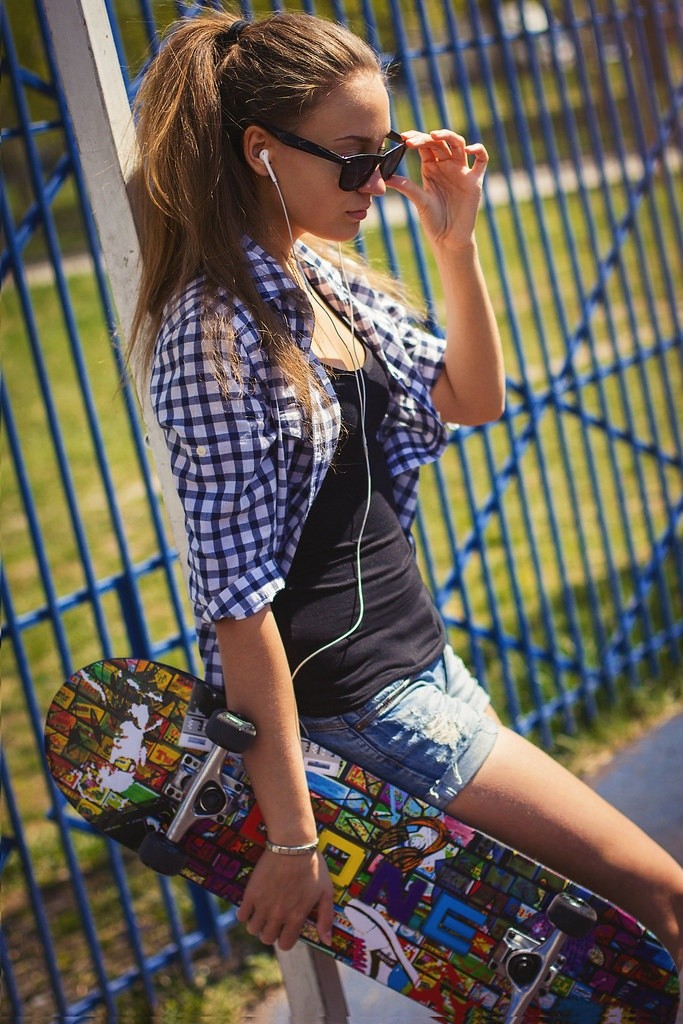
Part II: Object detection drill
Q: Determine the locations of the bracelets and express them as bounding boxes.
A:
[265,841,320,855]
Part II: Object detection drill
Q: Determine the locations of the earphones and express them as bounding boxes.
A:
[260,149,279,183]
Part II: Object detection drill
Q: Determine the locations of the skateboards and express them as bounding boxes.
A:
[36,650,683,1024]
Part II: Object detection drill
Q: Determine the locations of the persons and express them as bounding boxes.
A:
[124,13,683,968]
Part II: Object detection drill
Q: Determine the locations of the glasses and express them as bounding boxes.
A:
[252,117,409,191]
